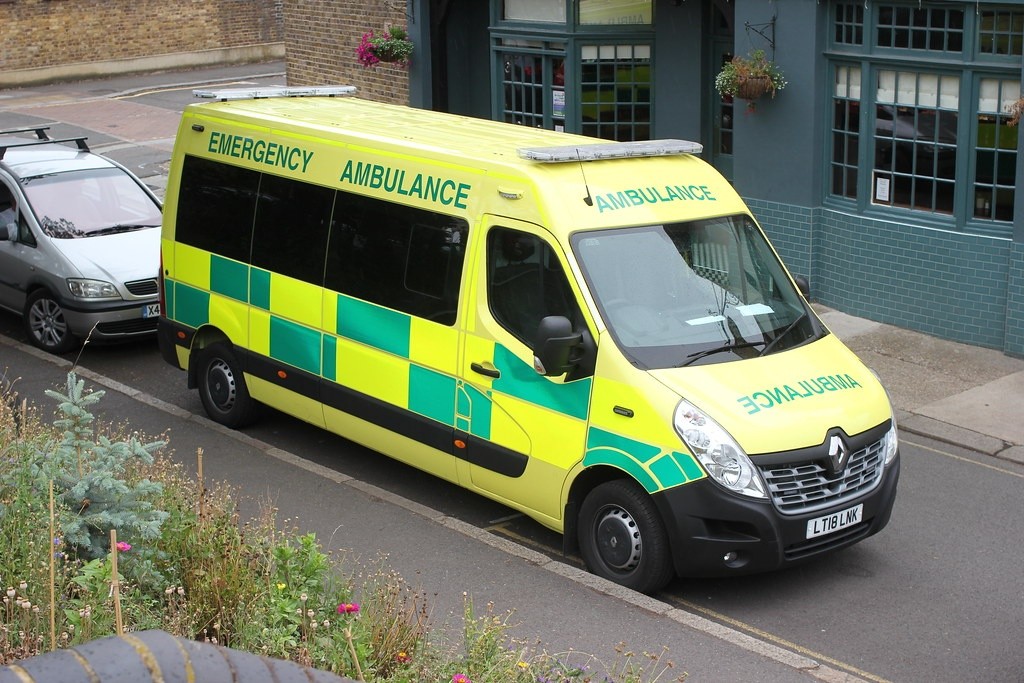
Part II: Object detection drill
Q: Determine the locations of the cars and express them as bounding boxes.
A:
[0,125,166,354]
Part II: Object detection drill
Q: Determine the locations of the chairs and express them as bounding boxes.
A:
[492,230,575,344]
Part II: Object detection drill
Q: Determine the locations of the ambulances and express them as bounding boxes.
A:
[156,83,902,596]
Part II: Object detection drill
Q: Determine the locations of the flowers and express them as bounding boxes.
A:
[714,50,789,100]
[355,26,415,68]
